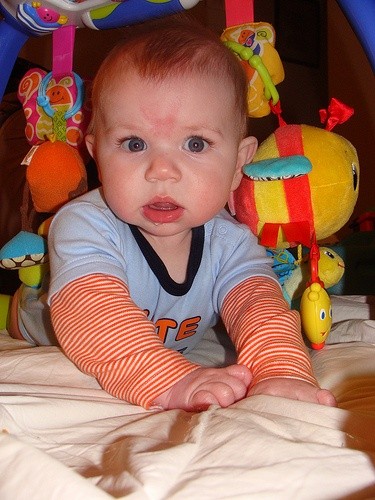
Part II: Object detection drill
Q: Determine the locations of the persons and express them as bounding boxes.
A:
[0,24,340,410]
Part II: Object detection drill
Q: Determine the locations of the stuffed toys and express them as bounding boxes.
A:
[265,245,345,309]
[1,231,49,288]
[21,137,92,235]
[300,284,336,350]
[231,94,361,351]
